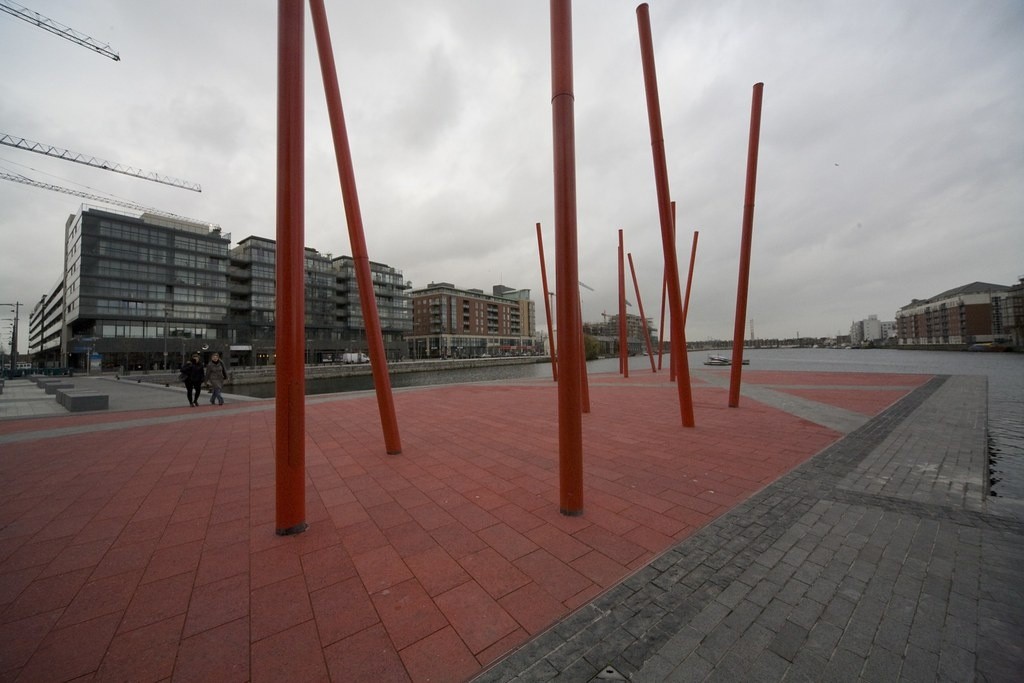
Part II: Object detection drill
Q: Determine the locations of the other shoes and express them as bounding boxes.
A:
[219,400,224,405]
[210,400,215,404]
[190,404,194,407]
[193,398,199,406]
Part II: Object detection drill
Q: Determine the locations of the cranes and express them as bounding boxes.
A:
[0,158,222,232]
[601,309,613,323]
[547,290,556,323]
[0,129,203,194]
[579,281,595,293]
[0,0,122,64]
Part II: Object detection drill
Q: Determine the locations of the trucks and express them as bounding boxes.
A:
[342,352,370,364]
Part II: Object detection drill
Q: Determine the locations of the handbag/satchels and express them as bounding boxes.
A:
[178,374,188,381]
[220,361,228,380]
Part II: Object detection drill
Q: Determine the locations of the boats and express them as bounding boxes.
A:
[703,355,750,366]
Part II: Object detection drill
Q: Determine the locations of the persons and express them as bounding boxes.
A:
[180,353,205,407]
[203,353,228,406]
